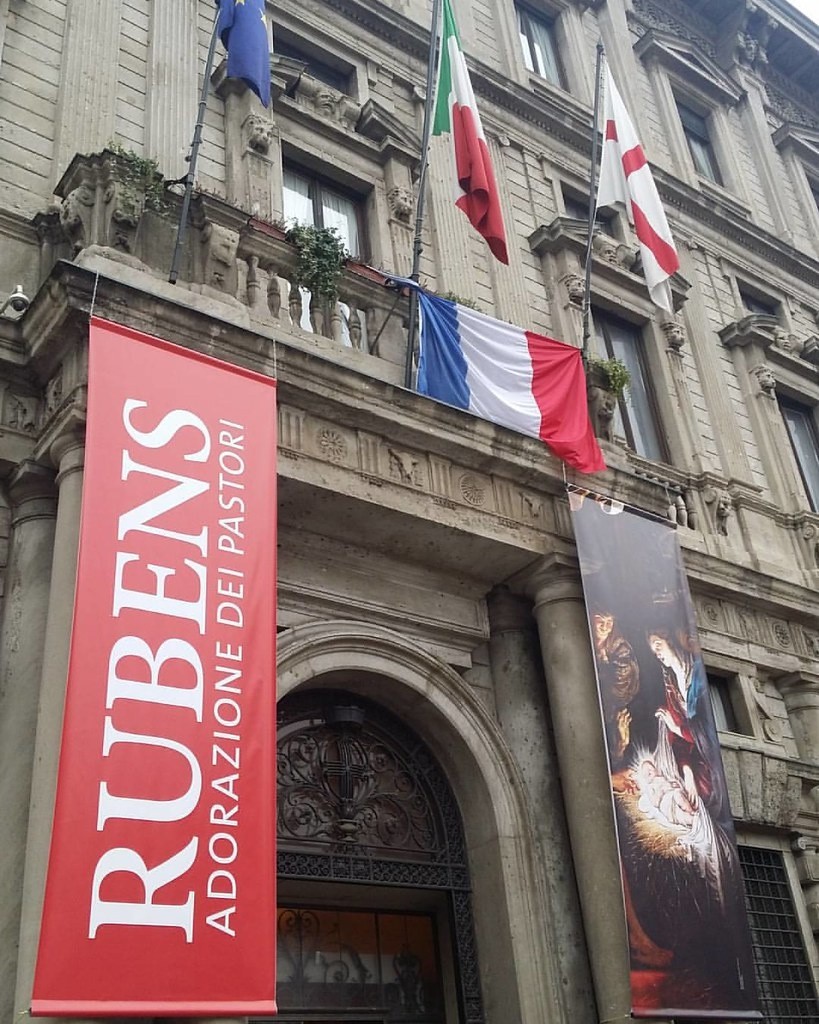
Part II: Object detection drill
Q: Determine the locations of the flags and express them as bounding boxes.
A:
[416,291,608,475]
[432,0,509,266]
[216,0,272,108]
[597,62,679,321]
